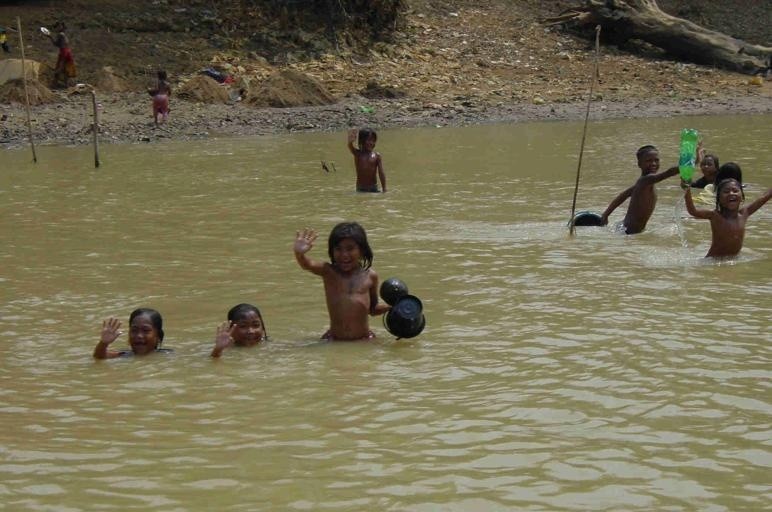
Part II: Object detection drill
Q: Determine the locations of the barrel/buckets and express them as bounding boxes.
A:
[383,294,426,341]
[567,210,607,227]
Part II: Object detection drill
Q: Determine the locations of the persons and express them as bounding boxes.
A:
[292,221,395,342]
[682,173,772,260]
[680,142,720,189]
[704,161,744,191]
[602,144,681,233]
[92,307,172,358]
[211,303,267,358]
[346,124,387,195]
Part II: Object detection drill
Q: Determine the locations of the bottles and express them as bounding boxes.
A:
[678,128,699,189]
